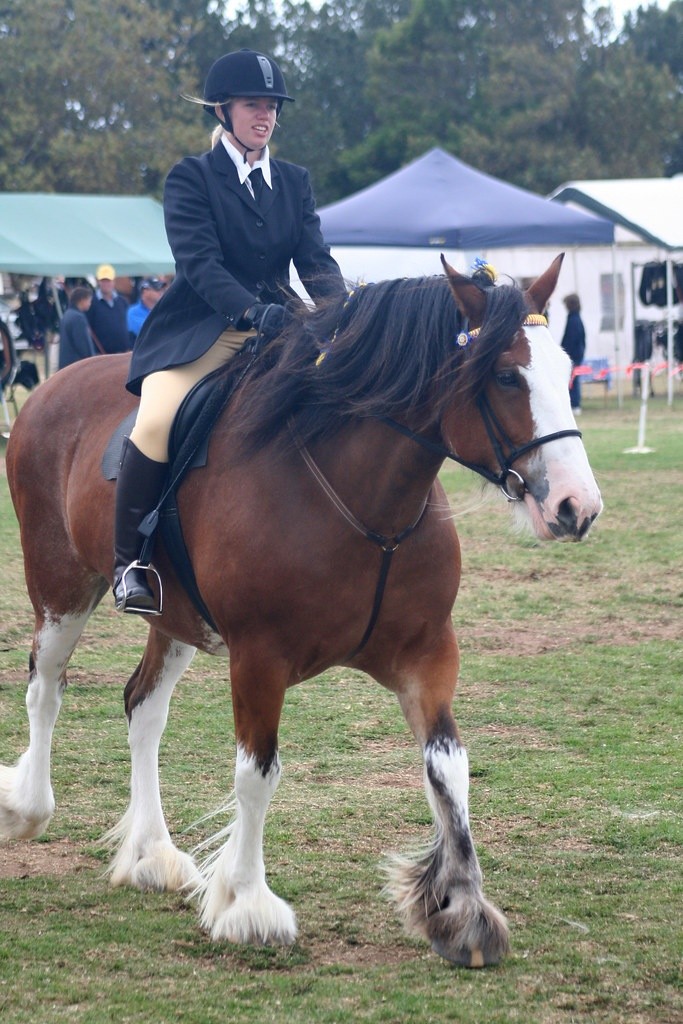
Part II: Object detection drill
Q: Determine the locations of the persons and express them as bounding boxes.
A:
[114,50,350,612]
[561,293,586,417]
[35,264,128,370]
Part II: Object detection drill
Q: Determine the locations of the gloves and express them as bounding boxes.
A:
[246,303,295,339]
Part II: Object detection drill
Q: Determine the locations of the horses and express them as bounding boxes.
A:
[1,249,605,971]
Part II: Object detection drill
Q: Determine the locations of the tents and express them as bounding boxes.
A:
[288,147,683,410]
[0,192,176,277]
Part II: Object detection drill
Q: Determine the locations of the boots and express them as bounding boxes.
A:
[111,434,173,615]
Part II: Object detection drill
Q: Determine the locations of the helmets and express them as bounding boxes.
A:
[203,48,295,115]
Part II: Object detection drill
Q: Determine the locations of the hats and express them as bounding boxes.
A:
[139,276,167,290]
[96,265,115,281]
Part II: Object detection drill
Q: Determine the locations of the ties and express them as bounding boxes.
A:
[248,168,263,205]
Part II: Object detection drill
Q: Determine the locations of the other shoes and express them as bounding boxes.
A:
[571,407,582,414]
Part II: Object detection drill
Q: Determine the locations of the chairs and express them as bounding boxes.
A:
[581,358,612,406]
